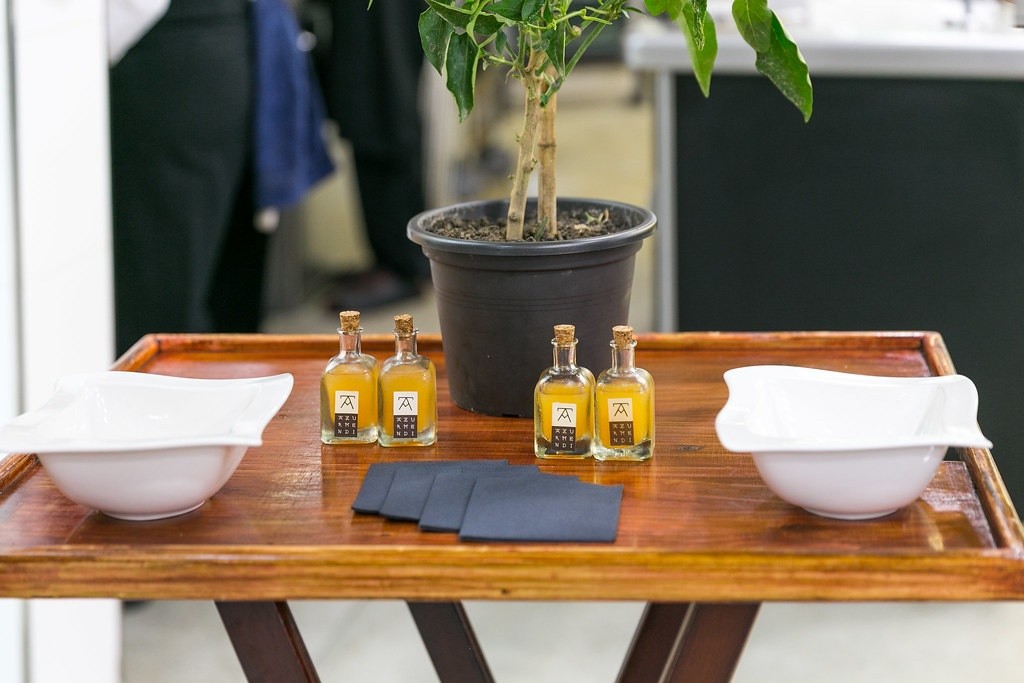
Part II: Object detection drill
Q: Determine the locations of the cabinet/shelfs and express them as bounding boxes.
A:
[624,1,1024,528]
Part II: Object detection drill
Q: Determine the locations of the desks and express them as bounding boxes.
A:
[1,330,1023,683]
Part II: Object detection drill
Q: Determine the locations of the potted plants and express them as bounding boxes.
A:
[364,0,812,421]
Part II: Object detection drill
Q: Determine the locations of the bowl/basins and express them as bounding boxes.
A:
[714,364,992,518]
[0,367,293,522]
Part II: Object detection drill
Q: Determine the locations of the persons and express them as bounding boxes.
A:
[297,1,431,315]
[105,0,282,612]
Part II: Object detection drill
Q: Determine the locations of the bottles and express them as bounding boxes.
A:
[322,309,378,446]
[379,316,437,448]
[594,324,655,465]
[534,323,594,461]
